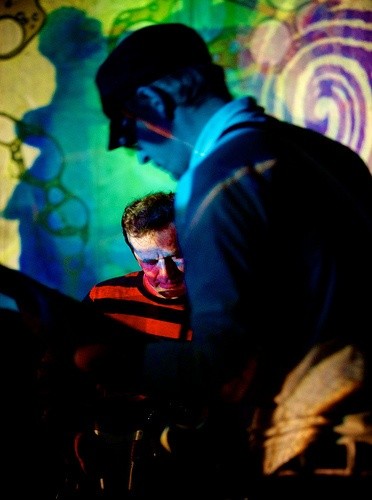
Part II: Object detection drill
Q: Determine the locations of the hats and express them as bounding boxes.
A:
[95,24,211,151]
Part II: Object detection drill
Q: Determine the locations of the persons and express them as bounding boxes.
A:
[66,189,257,417]
[96,23,372,433]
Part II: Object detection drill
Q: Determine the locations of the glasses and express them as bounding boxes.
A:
[118,103,149,147]
